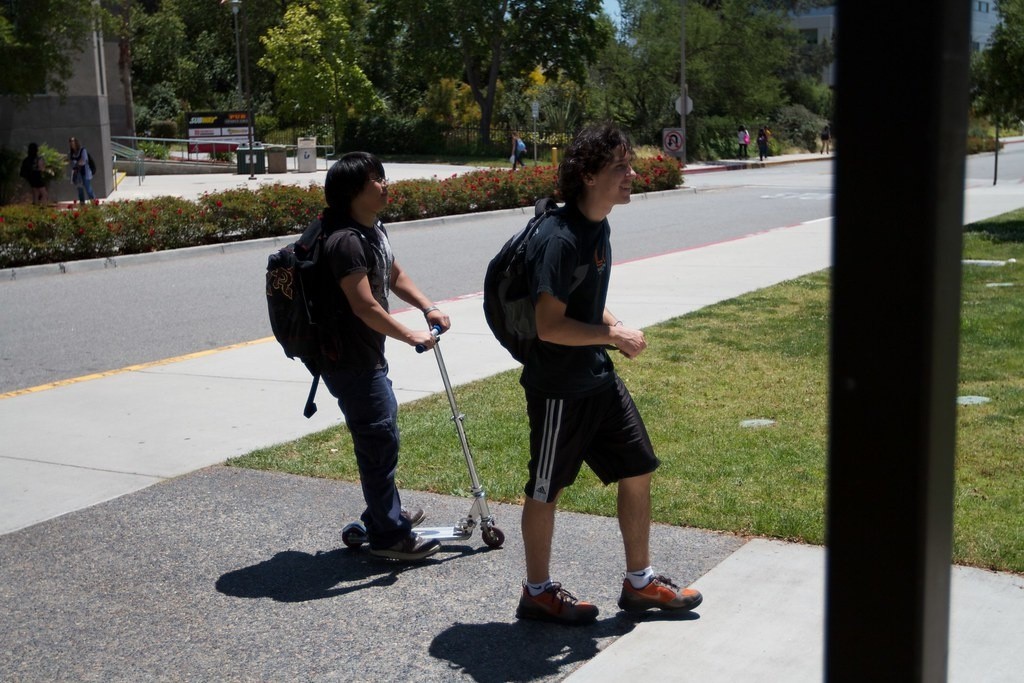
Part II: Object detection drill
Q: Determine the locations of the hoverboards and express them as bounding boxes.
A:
[339,322,506,562]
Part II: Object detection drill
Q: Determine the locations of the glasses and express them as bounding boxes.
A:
[367,177,389,188]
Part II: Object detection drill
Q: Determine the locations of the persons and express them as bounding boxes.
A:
[19,142,49,208]
[821,125,831,154]
[64,136,96,206]
[514,125,704,626]
[298,151,449,562]
[756,125,770,160]
[512,130,527,171]
[736,124,750,160]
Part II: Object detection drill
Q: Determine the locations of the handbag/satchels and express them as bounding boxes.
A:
[31,157,45,188]
[510,156,515,164]
[744,133,750,144]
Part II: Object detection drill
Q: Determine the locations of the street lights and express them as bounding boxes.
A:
[222,0,244,112]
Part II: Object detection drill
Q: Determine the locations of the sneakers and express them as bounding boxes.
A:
[515,578,599,625]
[618,571,703,614]
[368,532,442,560]
[364,506,426,529]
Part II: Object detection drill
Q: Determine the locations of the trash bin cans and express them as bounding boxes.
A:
[266,144,287,173]
[236,142,266,174]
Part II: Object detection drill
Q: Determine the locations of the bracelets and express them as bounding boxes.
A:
[424,306,440,317]
[614,320,624,327]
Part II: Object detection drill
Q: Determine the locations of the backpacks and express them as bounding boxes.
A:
[516,138,525,151]
[71,147,96,175]
[265,206,383,359]
[759,135,764,145]
[484,198,581,365]
